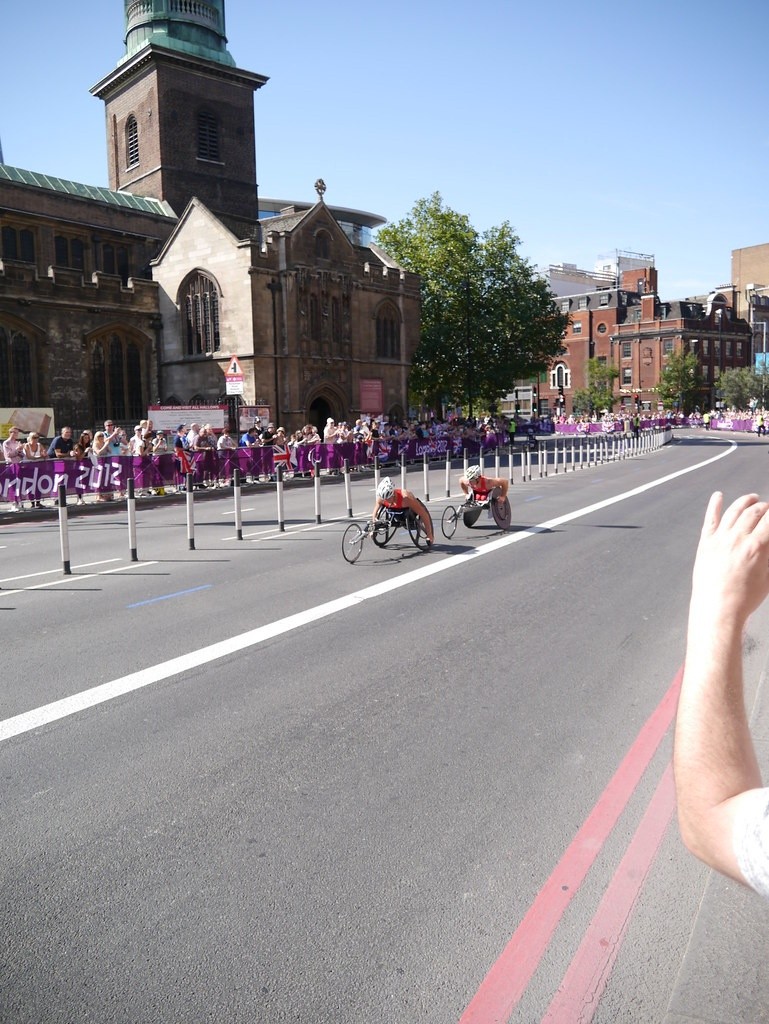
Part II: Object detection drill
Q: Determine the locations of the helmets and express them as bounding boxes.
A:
[377,476,395,500]
[465,465,481,481]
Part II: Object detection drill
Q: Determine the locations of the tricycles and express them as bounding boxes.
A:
[341,498,433,564]
[441,487,512,538]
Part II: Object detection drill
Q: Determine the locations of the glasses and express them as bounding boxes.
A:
[32,436,40,440]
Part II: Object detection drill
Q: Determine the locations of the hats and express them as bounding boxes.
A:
[156,430,163,435]
[198,476,275,493]
[275,427,285,434]
[267,423,274,428]
[176,423,188,433]
[254,416,261,423]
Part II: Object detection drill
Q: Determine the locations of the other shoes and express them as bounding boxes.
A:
[11,495,125,513]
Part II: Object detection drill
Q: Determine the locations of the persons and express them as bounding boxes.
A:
[702,412,711,431]
[507,418,517,446]
[320,413,506,476]
[711,405,769,434]
[671,491,769,900]
[757,411,767,437]
[370,475,435,546]
[22,432,47,509]
[175,416,323,491]
[458,466,508,506]
[688,406,702,429]
[2,426,27,512]
[517,413,597,450]
[48,420,168,505]
[599,410,684,440]
